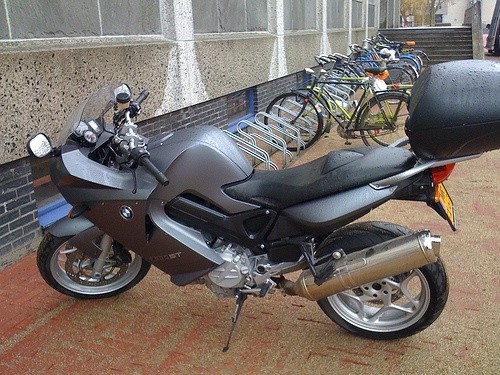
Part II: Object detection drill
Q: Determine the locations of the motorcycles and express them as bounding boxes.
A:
[27,60,500,342]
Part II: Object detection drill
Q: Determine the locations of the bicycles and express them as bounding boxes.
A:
[264,32,430,151]
